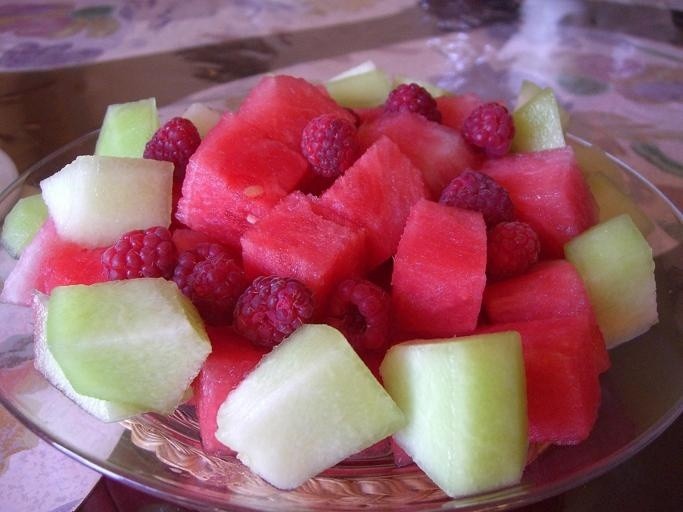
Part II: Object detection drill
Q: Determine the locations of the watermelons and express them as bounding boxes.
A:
[1,75,610,455]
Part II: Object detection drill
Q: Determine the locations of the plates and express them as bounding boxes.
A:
[1,88,683,510]
